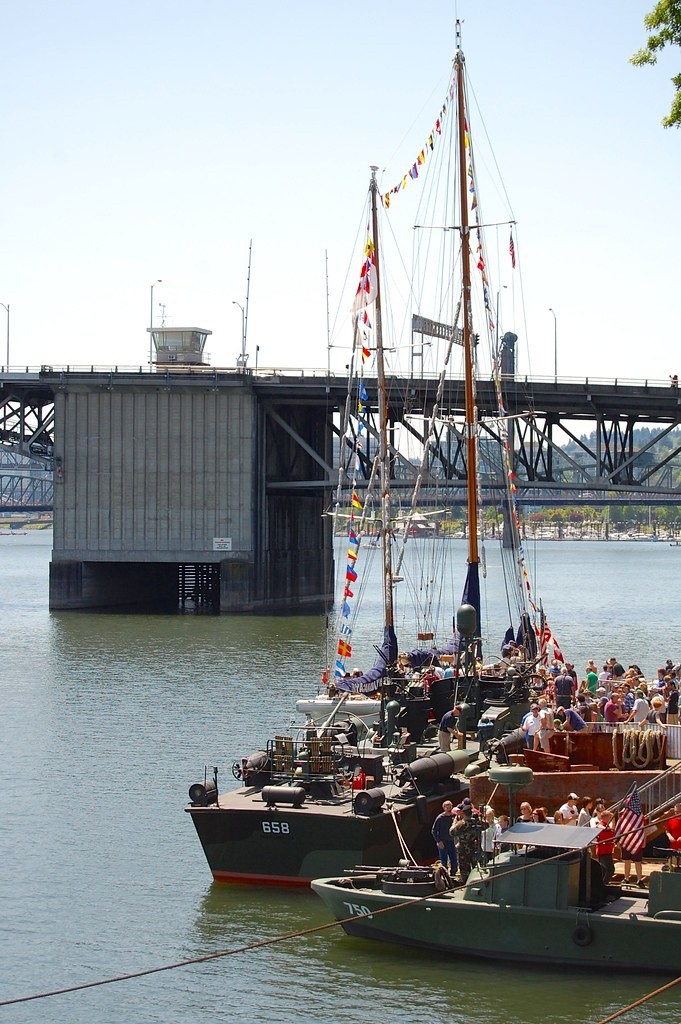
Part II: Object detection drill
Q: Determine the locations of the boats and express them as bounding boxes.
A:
[309,767,681,974]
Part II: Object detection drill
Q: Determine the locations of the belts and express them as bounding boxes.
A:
[557,695,570,698]
[545,729,554,730]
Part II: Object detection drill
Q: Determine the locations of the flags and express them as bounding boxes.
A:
[334,215,380,679]
[381,78,455,210]
[614,786,645,857]
[464,118,564,667]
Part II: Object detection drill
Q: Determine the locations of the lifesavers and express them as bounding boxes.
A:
[450,694,462,709]
[572,925,592,946]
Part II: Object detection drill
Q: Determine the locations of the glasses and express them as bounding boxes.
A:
[532,813,538,815]
[533,708,538,710]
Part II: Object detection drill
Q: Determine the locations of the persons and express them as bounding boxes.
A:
[534,657,652,725]
[664,803,681,869]
[520,698,588,754]
[431,792,648,888]
[639,700,666,728]
[501,640,530,675]
[438,705,462,751]
[639,659,681,724]
[397,654,483,692]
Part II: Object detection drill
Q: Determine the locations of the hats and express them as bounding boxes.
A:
[596,687,606,692]
[456,706,463,715]
[612,693,620,697]
[633,689,643,694]
[462,806,470,811]
[554,718,560,723]
[531,704,538,709]
[464,797,471,805]
[556,706,564,715]
[561,667,567,672]
[568,793,580,800]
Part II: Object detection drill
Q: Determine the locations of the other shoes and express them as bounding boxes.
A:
[623,878,629,884]
[636,880,646,888]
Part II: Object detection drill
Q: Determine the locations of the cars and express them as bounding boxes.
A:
[440,522,680,540]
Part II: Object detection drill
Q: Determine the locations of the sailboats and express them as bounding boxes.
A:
[184,20,680,891]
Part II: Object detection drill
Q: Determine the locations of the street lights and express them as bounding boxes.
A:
[231,301,244,375]
[150,279,162,373]
[549,308,557,383]
[496,285,507,382]
